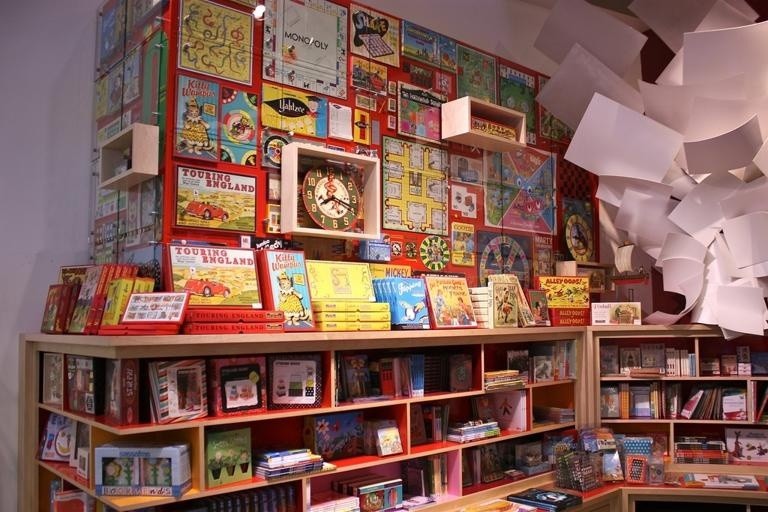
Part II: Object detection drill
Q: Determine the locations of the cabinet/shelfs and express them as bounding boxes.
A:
[15,323,768,509]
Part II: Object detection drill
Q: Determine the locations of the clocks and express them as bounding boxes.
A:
[302,165,360,227]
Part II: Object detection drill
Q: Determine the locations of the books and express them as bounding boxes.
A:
[253,447,452,512]
[509,487,583,510]
[617,343,768,425]
[400,352,528,444]
